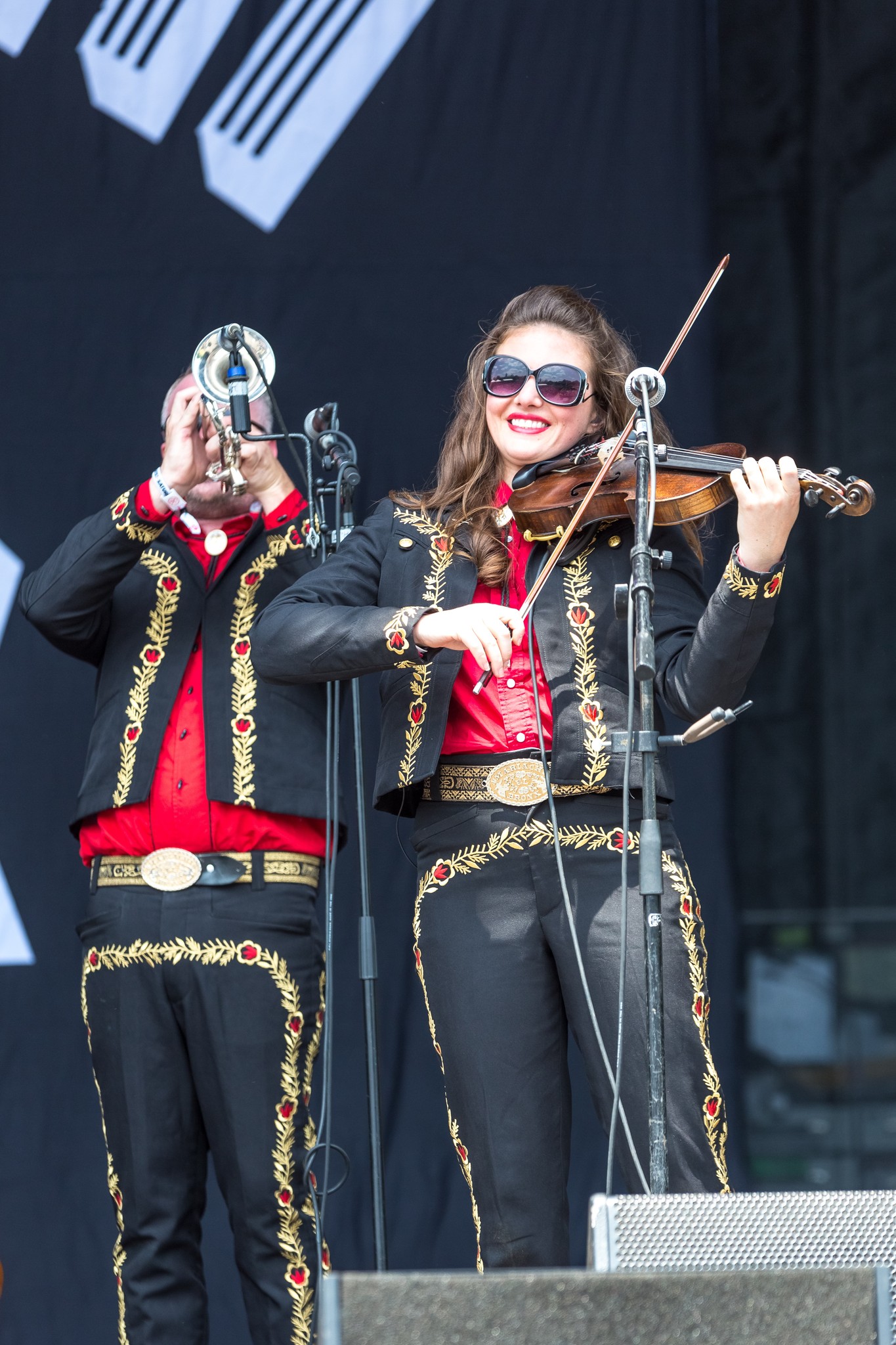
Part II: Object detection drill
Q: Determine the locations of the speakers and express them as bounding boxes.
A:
[312,1186,896,1345]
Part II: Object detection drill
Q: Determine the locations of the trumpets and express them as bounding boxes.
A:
[193,322,275,494]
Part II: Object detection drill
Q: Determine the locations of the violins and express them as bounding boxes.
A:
[507,429,875,538]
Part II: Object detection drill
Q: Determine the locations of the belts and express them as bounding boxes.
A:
[420,758,615,807]
[89,848,321,891]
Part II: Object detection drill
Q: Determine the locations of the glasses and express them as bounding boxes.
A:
[482,355,596,406]
[161,411,268,439]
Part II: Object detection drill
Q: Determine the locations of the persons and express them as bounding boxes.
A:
[16,371,342,1345]
[250,283,800,1274]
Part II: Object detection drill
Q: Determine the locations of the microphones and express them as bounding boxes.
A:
[304,402,340,442]
[218,322,244,353]
[625,366,666,407]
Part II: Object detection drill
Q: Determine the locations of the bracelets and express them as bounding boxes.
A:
[153,469,201,535]
